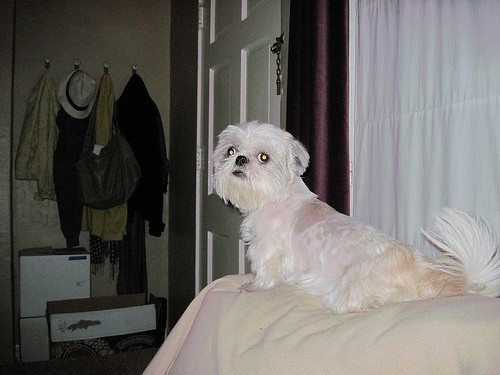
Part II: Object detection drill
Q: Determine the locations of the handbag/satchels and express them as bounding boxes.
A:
[76,133,141,210]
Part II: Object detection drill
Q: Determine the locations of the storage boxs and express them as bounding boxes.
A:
[45,290,160,341]
[17,246,92,317]
[19,316,50,365]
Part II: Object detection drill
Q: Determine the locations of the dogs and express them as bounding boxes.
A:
[210,120,499,315]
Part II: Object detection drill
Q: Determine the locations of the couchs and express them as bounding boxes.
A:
[142,267,499,374]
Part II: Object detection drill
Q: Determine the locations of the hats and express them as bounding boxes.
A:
[58,68,98,119]
